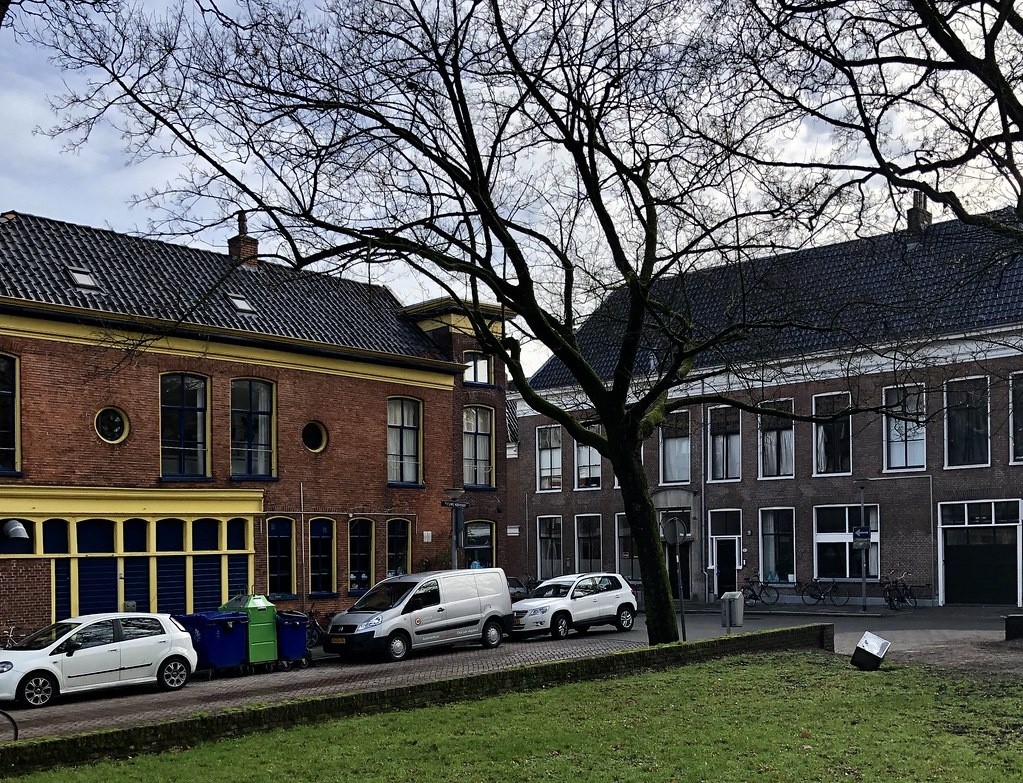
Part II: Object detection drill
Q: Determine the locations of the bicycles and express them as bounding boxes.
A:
[738,574,781,607]
[801,571,851,607]
[882,571,918,608]
[879,567,899,609]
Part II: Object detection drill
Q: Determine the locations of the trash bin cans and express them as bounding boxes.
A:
[276,609,309,671]
[174,609,249,681]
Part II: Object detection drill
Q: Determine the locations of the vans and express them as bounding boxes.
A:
[327,567,513,661]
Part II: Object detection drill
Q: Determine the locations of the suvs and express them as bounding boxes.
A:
[0,610,197,709]
[511,573,638,640]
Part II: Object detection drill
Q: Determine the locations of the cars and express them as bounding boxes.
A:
[506,577,528,595]
